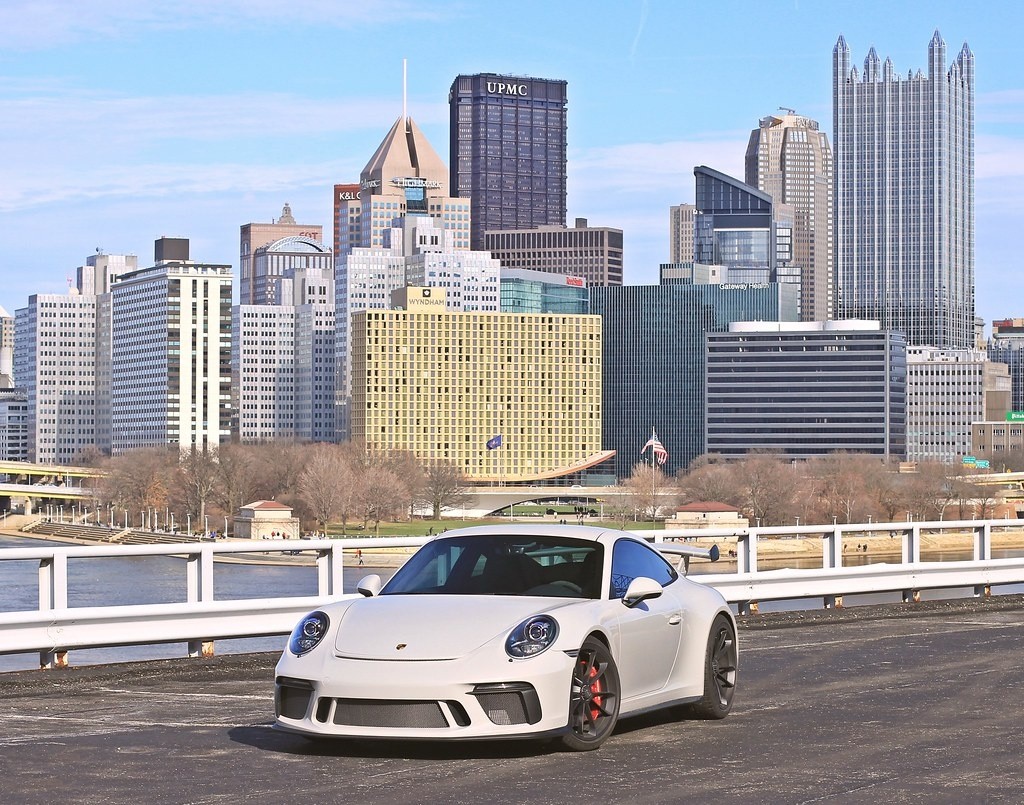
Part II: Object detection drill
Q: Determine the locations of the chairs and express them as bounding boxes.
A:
[481,550,542,595]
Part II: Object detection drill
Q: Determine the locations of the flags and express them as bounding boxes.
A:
[487,434,501,450]
[641,433,667,465]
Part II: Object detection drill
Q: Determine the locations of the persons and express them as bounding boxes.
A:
[560,519,566,524]
[263,530,289,540]
[357,549,364,566]
[211,531,216,538]
[309,529,325,537]
[151,525,154,532]
[430,526,447,536]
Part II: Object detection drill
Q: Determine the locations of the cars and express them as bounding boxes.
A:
[274,522,738,752]
